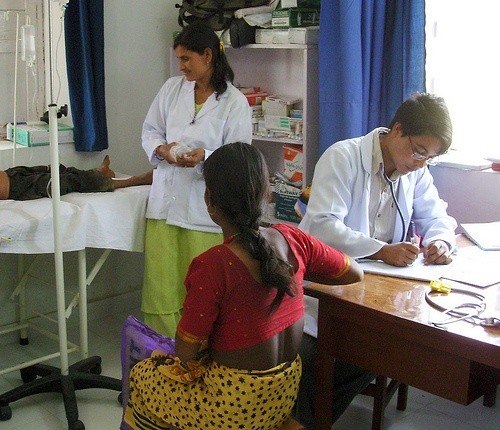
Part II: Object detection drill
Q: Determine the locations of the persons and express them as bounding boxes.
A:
[141,25,255,355]
[1,154,156,202]
[115,143,369,430]
[284,92,454,429]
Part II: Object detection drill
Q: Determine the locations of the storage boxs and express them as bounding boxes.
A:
[2,121,74,147]
[255,0,321,46]
[244,93,303,135]
[283,146,303,182]
[274,182,303,223]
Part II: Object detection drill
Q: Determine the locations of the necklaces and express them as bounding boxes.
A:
[191,87,206,126]
[223,231,243,244]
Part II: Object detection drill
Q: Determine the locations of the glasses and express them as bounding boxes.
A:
[408,134,439,166]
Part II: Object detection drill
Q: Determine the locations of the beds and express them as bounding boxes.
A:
[0,172,152,377]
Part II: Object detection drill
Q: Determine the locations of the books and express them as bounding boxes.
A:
[459,220,500,251]
[358,251,500,289]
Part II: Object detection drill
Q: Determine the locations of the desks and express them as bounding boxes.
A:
[302,232,500,430]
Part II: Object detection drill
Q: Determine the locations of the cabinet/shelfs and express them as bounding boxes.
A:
[168,44,318,227]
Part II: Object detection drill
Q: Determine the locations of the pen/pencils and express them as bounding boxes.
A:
[409,221,415,246]
